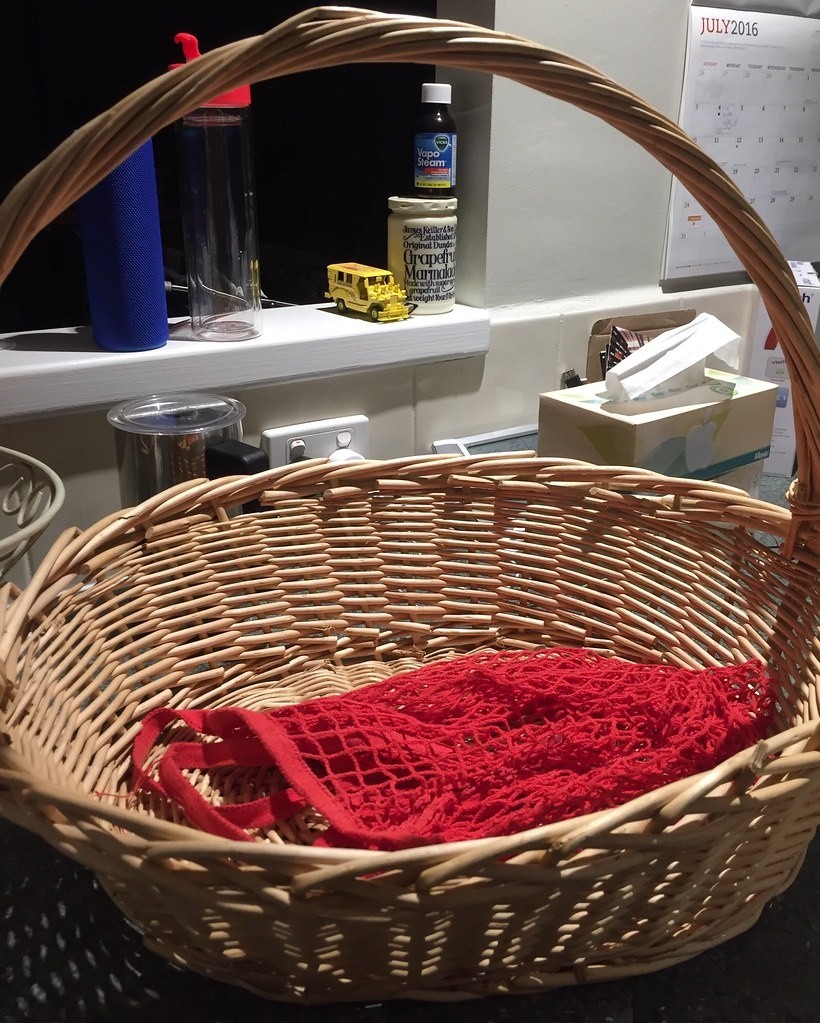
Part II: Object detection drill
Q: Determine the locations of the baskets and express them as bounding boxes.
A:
[0,7,819,998]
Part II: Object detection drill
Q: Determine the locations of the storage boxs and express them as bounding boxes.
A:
[746,260,820,482]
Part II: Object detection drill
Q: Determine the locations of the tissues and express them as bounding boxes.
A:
[536,310,781,498]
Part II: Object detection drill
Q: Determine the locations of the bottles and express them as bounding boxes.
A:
[164,32,265,343]
[411,80,457,199]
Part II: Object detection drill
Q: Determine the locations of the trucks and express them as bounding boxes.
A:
[324,262,411,322]
